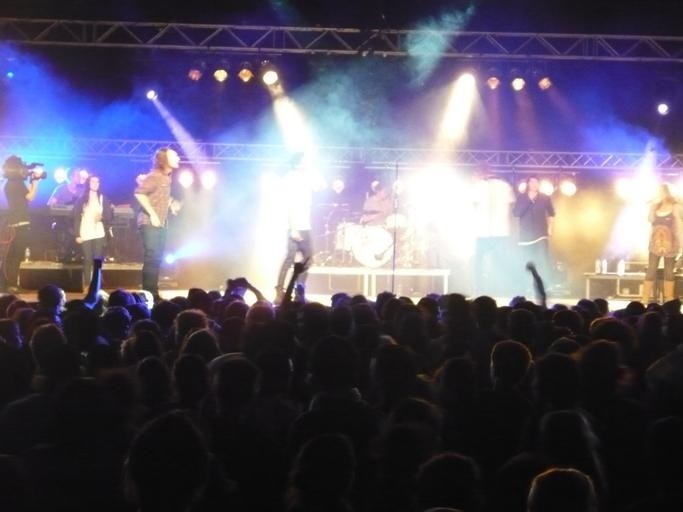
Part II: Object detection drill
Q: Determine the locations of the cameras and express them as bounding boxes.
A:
[27,163,46,182]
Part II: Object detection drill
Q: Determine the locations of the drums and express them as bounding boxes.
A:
[387,213,409,233]
[353,226,394,269]
[335,221,358,249]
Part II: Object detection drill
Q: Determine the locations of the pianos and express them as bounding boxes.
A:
[39,205,133,219]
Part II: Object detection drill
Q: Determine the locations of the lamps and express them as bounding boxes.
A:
[479,52,551,93]
[187,43,279,89]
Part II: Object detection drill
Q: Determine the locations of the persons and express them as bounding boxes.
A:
[275,185,316,305]
[73,175,116,294]
[133,147,181,304]
[361,179,393,224]
[2,155,45,295]
[639,184,683,306]
[1,255,682,511]
[514,175,556,284]
[47,165,89,262]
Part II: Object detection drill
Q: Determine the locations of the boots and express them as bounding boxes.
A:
[663,280,675,303]
[640,280,654,305]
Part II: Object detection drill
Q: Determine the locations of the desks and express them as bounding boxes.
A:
[291,264,449,302]
[17,257,145,293]
[581,272,664,307]
